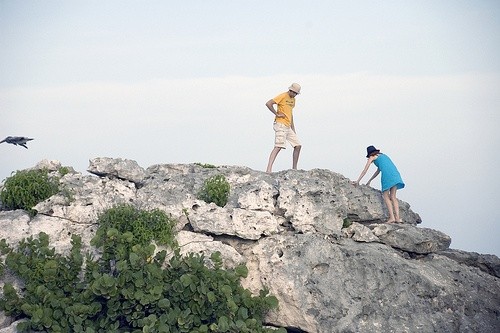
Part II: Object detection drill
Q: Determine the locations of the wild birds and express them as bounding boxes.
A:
[0,136,34,149]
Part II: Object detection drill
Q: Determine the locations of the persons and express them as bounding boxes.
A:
[352,146,404,223]
[266,83,301,172]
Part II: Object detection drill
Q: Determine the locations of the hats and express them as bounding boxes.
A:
[289,82,300,94]
[366,146,380,158]
